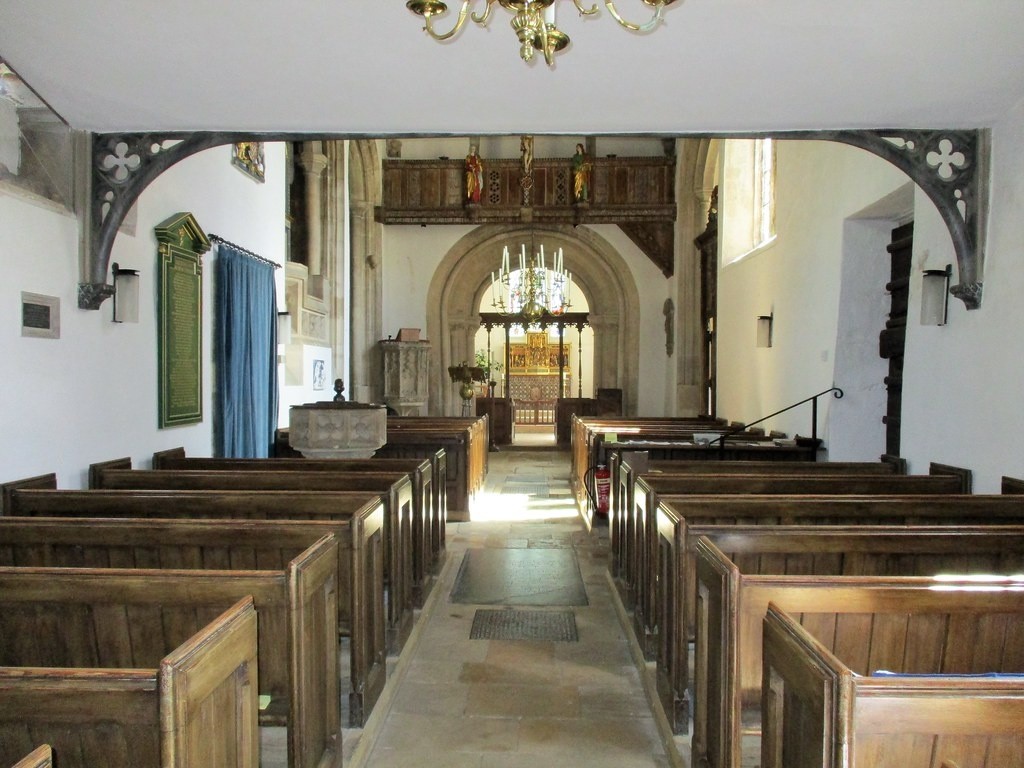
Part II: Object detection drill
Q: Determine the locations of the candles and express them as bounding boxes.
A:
[502,373,504,379]
[563,373,566,379]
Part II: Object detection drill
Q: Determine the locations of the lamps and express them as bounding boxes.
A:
[112,263,141,324]
[919,264,956,326]
[277,311,292,344]
[755,315,772,348]
[406,0,675,66]
[490,223,590,334]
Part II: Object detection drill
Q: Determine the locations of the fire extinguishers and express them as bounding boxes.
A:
[583,463,611,518]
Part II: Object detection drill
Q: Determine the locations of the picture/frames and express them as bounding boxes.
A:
[231,140,266,184]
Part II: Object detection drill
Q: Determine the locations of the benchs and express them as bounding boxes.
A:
[568,396,1024,768]
[0,413,491,768]
